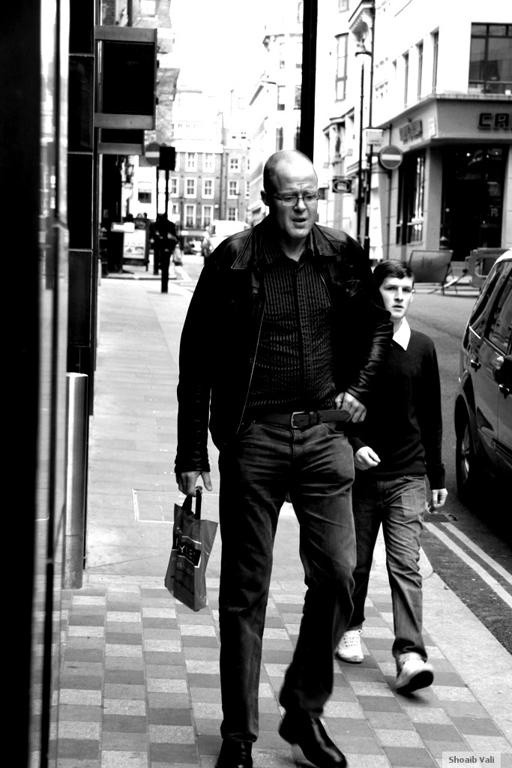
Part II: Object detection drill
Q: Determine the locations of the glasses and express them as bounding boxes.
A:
[273,192,319,206]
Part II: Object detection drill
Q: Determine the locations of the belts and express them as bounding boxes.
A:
[258,408,350,429]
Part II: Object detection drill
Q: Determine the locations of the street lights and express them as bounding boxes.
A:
[355,39,372,245]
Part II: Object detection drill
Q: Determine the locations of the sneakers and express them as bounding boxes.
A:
[334,629,364,663]
[393,652,435,695]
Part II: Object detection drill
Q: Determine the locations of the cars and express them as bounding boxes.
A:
[201,220,252,257]
[454,252,512,514]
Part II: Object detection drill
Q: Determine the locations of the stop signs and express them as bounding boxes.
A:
[144,143,161,166]
[380,144,404,170]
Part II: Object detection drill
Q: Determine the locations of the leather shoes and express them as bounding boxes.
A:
[215,739,252,768]
[279,711,347,768]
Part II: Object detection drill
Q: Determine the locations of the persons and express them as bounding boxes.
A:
[173,146,397,765]
[122,210,178,270]
[335,254,448,691]
